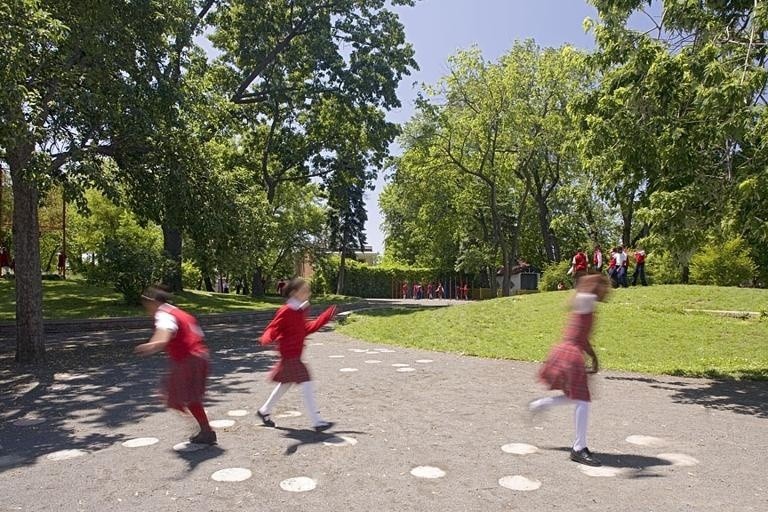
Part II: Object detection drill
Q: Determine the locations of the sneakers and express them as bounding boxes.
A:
[570,446,601,467]
[190,430,216,445]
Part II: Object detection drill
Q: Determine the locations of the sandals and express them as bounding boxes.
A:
[256,410,276,428]
[315,422,335,432]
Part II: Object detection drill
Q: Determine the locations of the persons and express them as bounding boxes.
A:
[134,290,217,445]
[208,277,248,294]
[261,277,266,294]
[529,274,610,467]
[402,279,469,300]
[59,250,68,274]
[0,247,16,278]
[277,279,285,296]
[257,279,335,432]
[556,242,648,289]
